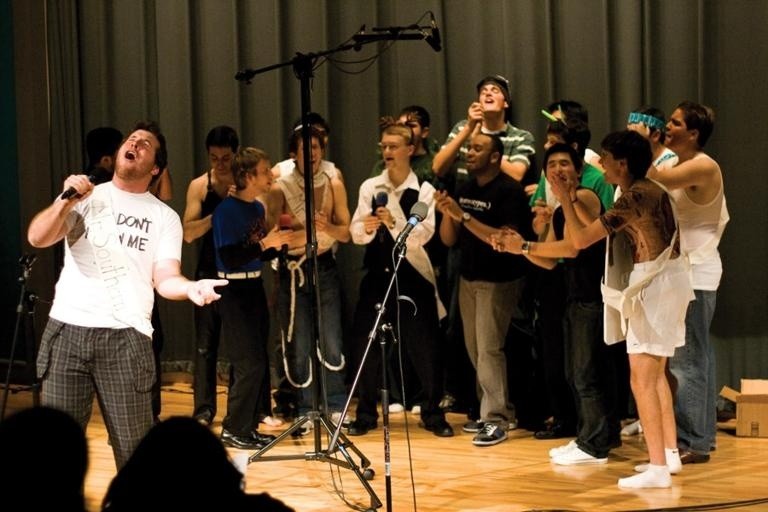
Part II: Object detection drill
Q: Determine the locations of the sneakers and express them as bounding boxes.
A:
[621,419,641,436]
[617,463,671,489]
[381,405,454,438]
[290,415,314,437]
[331,412,353,431]
[634,449,683,474]
[347,415,379,436]
[549,439,608,465]
[462,418,517,444]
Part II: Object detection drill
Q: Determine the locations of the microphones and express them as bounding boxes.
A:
[392,199,428,252]
[374,192,388,212]
[279,212,291,270]
[419,26,442,52]
[427,15,442,42]
[59,164,109,203]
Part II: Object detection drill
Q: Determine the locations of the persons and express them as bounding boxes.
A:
[101,416,293,512]
[26,118,230,474]
[182,76,729,489]
[83,123,174,425]
[0,406,88,512]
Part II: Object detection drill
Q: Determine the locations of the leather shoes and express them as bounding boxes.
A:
[219,425,277,450]
[677,444,716,464]
[536,423,578,439]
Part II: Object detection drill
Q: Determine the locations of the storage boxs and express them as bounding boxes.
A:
[715,372,767,441]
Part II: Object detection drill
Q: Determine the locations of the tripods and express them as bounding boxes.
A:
[245,87,382,510]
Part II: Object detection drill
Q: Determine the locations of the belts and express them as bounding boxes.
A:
[216,270,263,280]
